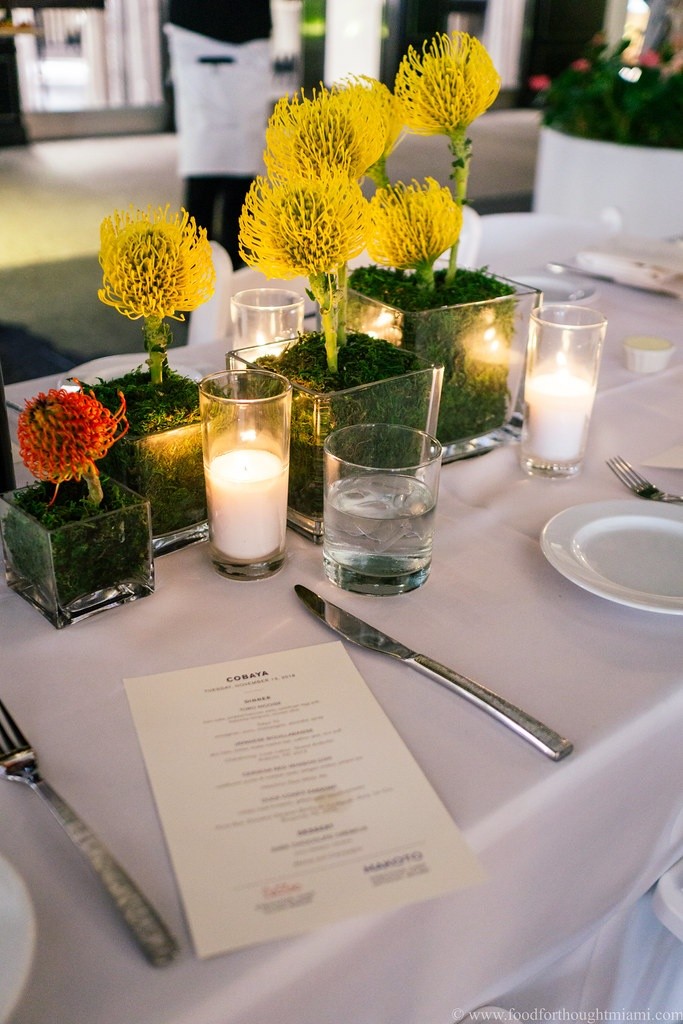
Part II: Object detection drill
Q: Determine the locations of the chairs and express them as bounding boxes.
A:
[188,263,313,345]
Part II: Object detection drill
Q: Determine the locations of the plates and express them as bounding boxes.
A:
[0,857,37,1024]
[539,499,683,615]
[56,353,205,391]
[503,274,602,306]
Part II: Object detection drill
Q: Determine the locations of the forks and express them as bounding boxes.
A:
[0,699,180,972]
[604,454,683,505]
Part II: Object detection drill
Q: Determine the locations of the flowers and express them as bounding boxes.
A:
[15,30,499,504]
[524,28,683,150]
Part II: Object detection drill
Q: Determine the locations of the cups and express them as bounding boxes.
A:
[197,369,293,582]
[230,287,304,370]
[518,303,608,482]
[323,423,445,597]
[621,334,675,373]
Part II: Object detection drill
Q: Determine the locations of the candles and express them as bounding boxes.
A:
[525,378,591,461]
[204,450,289,558]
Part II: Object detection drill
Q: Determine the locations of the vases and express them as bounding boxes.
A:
[224,329,446,542]
[78,371,212,559]
[0,472,155,631]
[529,122,683,237]
[341,257,545,467]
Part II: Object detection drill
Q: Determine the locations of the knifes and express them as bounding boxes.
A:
[295,584,573,765]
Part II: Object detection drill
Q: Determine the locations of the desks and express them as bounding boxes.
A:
[1,237,683,1024]
